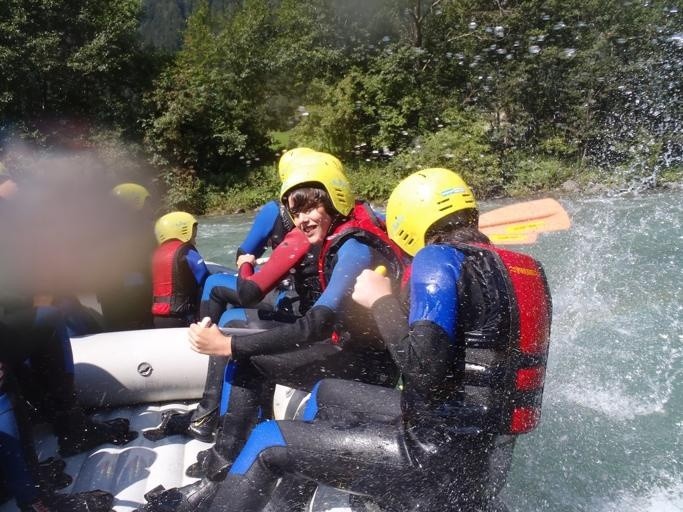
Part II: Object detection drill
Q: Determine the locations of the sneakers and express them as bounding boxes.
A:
[58,418,130,457]
[163,409,217,443]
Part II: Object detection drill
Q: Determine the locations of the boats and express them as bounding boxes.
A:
[0,260,381,512]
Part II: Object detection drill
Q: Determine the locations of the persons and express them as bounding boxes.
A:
[1,147,553,512]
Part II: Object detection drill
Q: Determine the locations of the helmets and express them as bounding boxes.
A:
[385,166,479,259]
[107,180,156,213]
[151,209,199,246]
[277,146,355,218]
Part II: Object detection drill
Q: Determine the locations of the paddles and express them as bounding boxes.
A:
[479,198,572,235]
[254,233,539,265]
